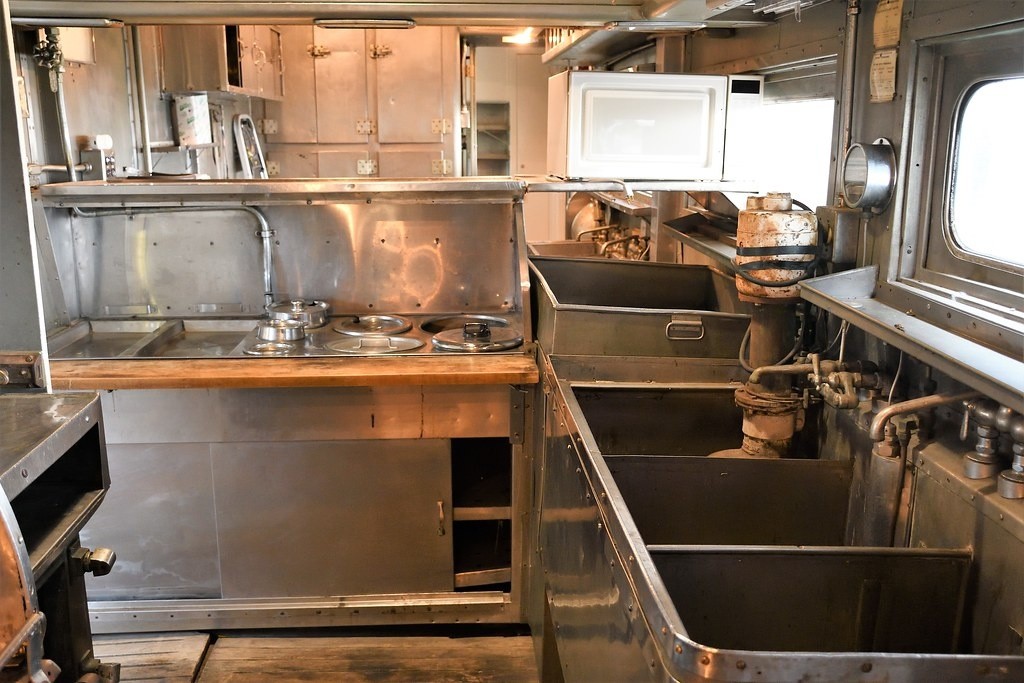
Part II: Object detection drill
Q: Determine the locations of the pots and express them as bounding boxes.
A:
[256,316,306,342]
[264,296,331,329]
[328,313,425,354]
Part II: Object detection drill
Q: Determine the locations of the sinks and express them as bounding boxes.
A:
[559,371,861,461]
[46,311,181,361]
[594,444,974,552]
[638,538,1024,670]
[121,314,264,369]
[525,240,622,262]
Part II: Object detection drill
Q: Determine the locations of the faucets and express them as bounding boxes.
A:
[869,386,984,443]
[599,234,637,257]
[575,222,620,242]
[748,362,812,384]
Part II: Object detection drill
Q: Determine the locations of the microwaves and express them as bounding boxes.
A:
[545,69,764,182]
